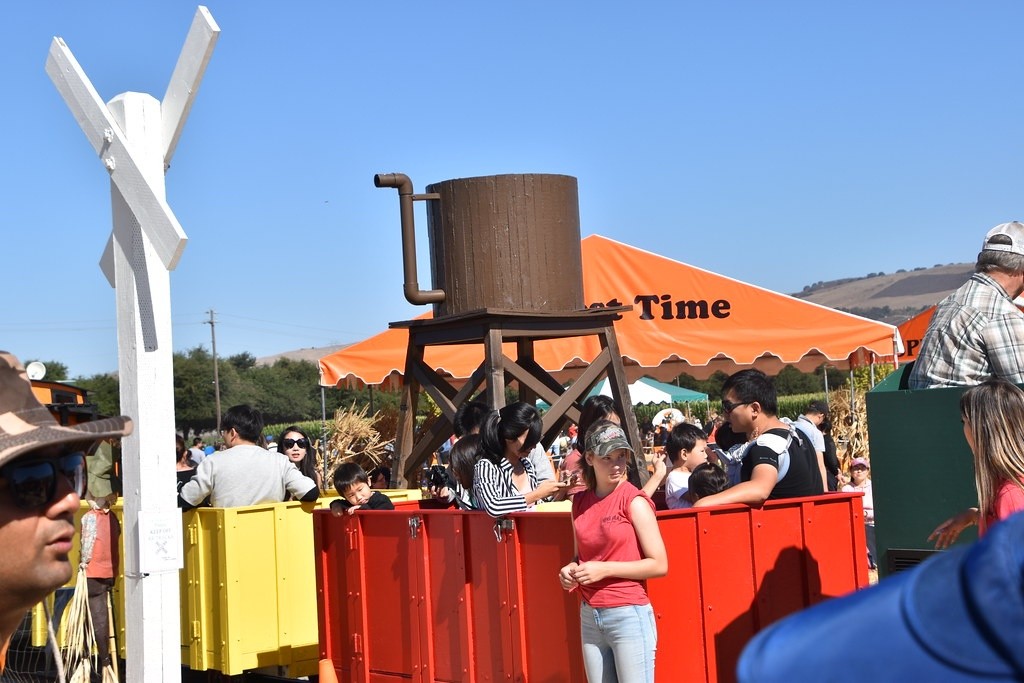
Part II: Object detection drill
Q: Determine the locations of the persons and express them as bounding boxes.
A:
[559,419,669,683]
[738,509,1024,683]
[0,351,133,683]
[927,379,1024,550]
[87,405,395,517]
[416,367,878,569]
[906,219,1024,389]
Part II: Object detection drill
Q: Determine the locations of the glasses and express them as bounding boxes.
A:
[284,438,306,449]
[220,428,225,436]
[723,399,751,413]
[854,464,867,471]
[0,450,86,516]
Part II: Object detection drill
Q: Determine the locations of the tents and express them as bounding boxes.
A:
[895,278,1024,361]
[317,235,903,488]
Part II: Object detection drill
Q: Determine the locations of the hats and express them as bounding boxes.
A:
[811,401,829,415]
[850,458,867,467]
[0,351,132,469]
[583,421,636,458]
[982,220,1024,255]
